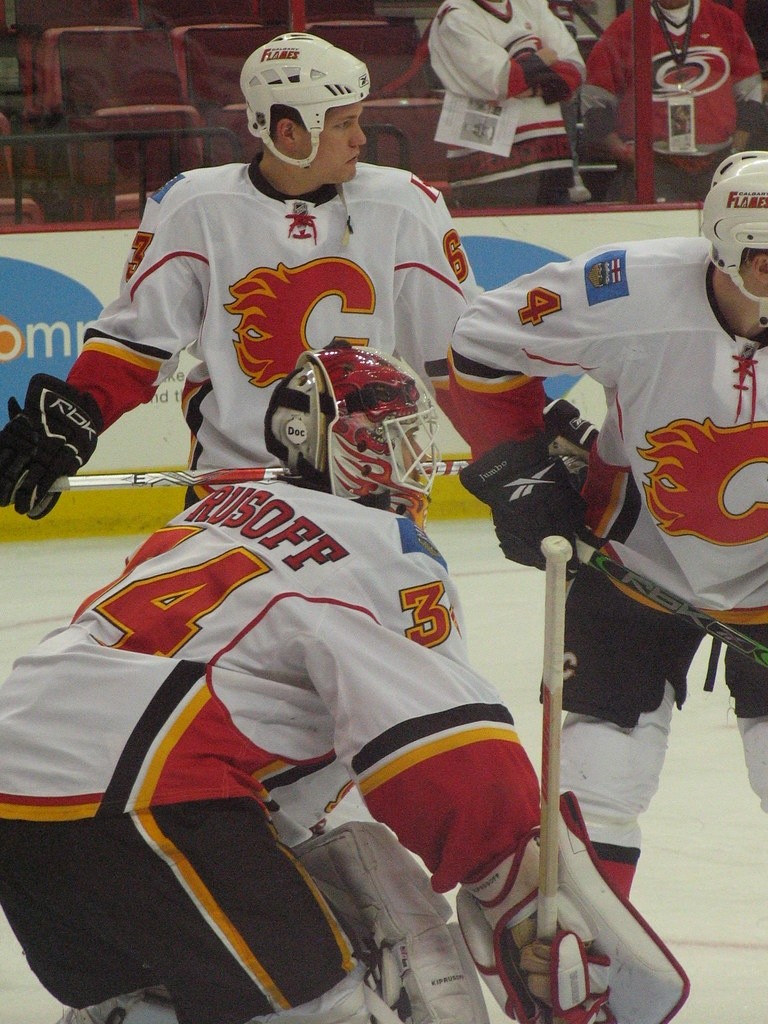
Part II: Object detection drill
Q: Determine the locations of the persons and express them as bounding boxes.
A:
[0,340,620,1023]
[421,1,590,208]
[446,151,768,908]
[578,0,763,202]
[0,27,598,529]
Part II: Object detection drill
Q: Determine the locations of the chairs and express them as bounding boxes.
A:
[0,0,459,228]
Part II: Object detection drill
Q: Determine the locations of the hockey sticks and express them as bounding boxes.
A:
[45,454,473,496]
[533,532,574,947]
[575,537,768,669]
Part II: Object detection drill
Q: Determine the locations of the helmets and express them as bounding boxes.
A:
[239,32,370,167]
[264,347,447,511]
[701,150,768,287]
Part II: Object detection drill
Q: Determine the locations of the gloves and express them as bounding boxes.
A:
[543,398,599,449]
[511,917,567,1024]
[0,372,104,520]
[459,433,588,581]
